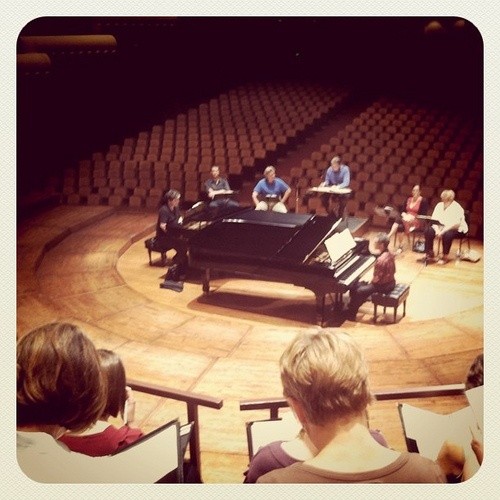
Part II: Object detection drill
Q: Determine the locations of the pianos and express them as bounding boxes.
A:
[169,204,378,329]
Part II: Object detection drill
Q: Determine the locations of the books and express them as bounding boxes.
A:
[246,418,288,465]
[178,420,195,466]
[397,401,481,483]
[110,417,179,484]
[464,386,484,432]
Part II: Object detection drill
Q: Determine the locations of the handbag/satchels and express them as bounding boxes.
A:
[413,240,425,252]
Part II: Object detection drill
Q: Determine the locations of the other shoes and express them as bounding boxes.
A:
[416,258,435,263]
[437,259,448,265]
[397,248,402,254]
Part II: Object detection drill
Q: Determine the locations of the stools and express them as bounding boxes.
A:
[144,238,170,266]
[372,284,411,324]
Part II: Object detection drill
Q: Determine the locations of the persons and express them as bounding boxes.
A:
[340,231,396,322]
[252,166,292,212]
[319,156,350,218]
[156,189,191,264]
[243,329,484,484]
[422,189,468,264]
[204,165,230,200]
[16,322,147,484]
[387,184,428,254]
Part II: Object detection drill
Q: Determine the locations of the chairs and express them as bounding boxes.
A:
[17,68,484,257]
